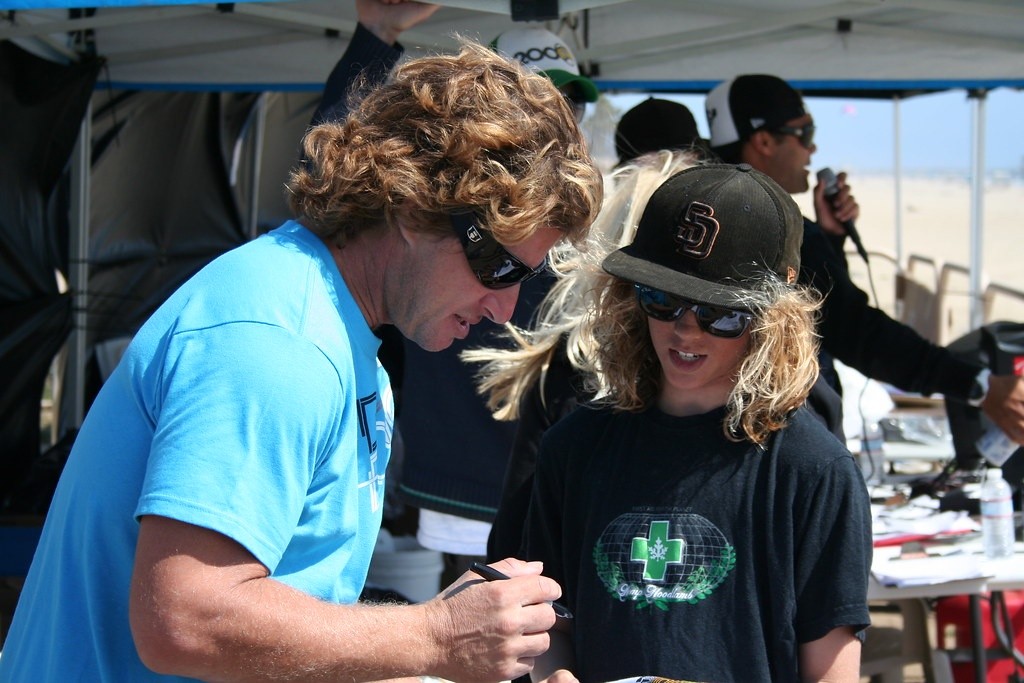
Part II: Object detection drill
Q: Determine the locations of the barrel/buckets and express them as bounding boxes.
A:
[365,535,444,602]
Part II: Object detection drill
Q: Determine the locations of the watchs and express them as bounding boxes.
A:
[967,368,992,407]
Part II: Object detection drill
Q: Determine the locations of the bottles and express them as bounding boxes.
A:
[861,415,884,486]
[980,468,1016,561]
[975,424,1021,467]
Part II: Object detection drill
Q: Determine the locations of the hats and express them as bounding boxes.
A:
[611,96,711,171]
[601,163,804,309]
[487,27,599,102]
[705,75,804,163]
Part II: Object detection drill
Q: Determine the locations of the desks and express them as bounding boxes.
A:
[867,503,1024,683]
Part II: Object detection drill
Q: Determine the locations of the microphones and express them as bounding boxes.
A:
[818,166,869,264]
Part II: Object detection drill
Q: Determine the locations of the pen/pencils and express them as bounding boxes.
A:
[468,561,574,620]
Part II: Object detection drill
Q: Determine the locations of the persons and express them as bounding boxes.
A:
[0,35,602,683]
[298,0,1024,591]
[515,163,871,683]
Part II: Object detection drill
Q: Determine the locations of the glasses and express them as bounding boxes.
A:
[639,285,765,338]
[768,125,815,149]
[450,215,550,290]
[563,97,585,123]
